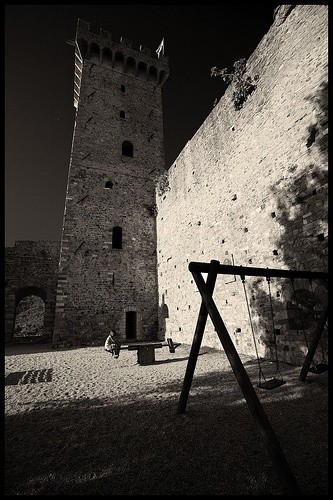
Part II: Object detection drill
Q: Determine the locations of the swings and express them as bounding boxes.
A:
[292,283,327,374]
[242,280,286,390]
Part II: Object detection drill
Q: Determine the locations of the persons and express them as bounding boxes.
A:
[104,330,120,358]
